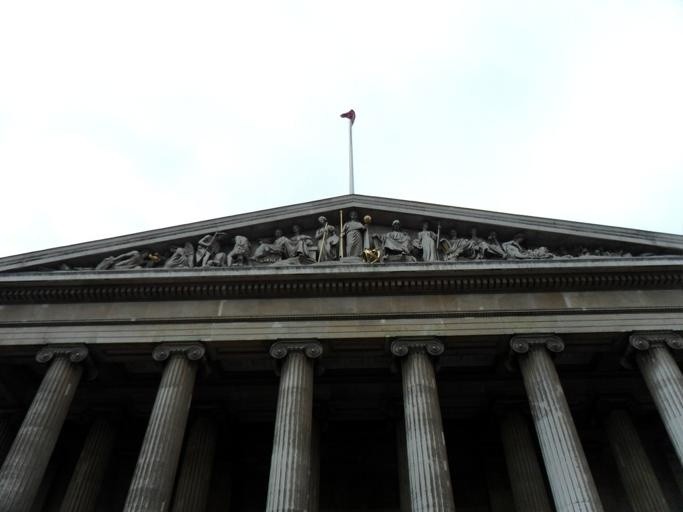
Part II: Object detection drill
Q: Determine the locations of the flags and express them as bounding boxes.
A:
[339,109,354,125]
